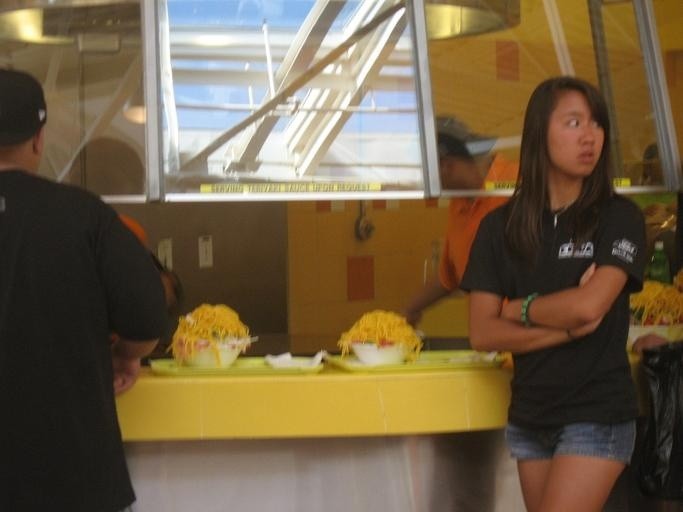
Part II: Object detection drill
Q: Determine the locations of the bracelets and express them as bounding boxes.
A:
[519,290,539,328]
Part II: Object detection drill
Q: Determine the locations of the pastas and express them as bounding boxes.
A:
[334,308,425,362]
[630,272,683,329]
[165,303,253,370]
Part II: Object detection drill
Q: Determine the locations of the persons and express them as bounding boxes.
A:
[117,208,177,320]
[403,113,521,327]
[455,71,652,509]
[71,134,146,200]
[0,66,179,512]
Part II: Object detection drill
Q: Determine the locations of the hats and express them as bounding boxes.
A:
[0,69,47,147]
[435,116,497,164]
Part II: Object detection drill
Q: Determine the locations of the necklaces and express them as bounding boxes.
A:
[548,196,578,228]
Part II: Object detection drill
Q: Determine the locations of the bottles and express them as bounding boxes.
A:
[648,239,672,287]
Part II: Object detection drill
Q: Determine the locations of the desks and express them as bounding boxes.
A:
[116,365,511,512]
[411,321,683,512]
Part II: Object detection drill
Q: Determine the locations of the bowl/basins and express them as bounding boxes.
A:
[177,338,247,370]
[349,339,411,368]
